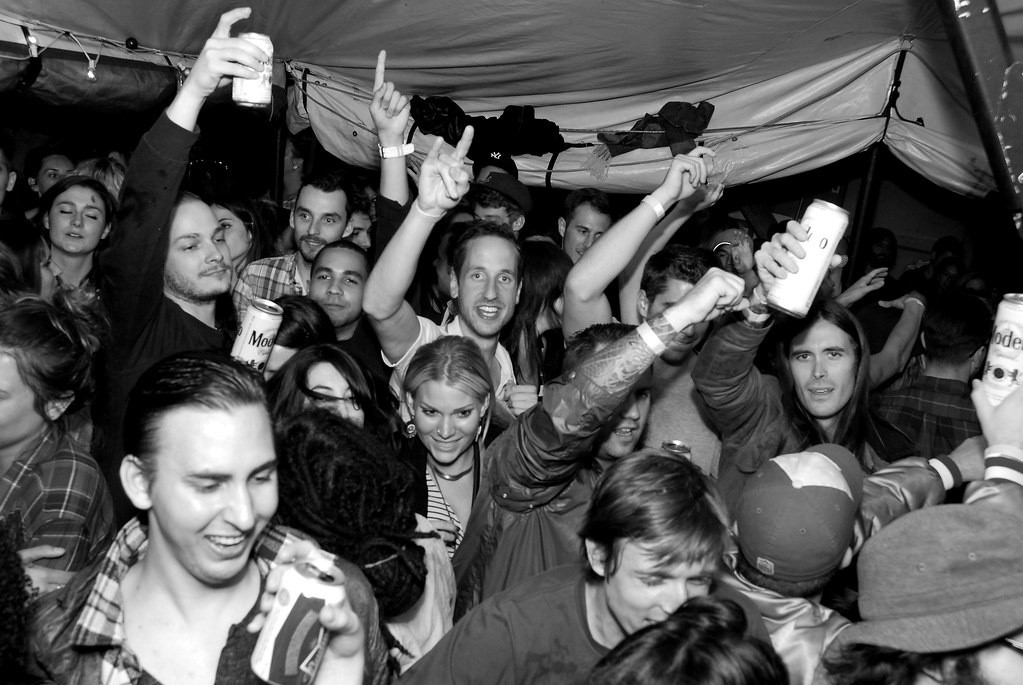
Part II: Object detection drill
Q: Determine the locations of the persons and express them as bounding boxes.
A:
[0,8,1023,685]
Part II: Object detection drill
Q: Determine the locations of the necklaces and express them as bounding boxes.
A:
[429,459,473,481]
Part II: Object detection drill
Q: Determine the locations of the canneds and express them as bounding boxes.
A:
[982,293,1023,405]
[230,297,284,374]
[765,198,849,319]
[232,31,272,108]
[659,439,692,462]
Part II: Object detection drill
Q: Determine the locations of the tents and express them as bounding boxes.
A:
[0,0,1023,229]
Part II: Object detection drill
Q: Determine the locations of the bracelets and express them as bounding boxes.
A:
[377,139,415,160]
[416,198,441,218]
[636,321,668,357]
[904,297,926,311]
[641,195,666,224]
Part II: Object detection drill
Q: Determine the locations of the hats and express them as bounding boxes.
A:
[471,150,520,180]
[822,503,1023,671]
[734,443,863,581]
[708,228,753,254]
